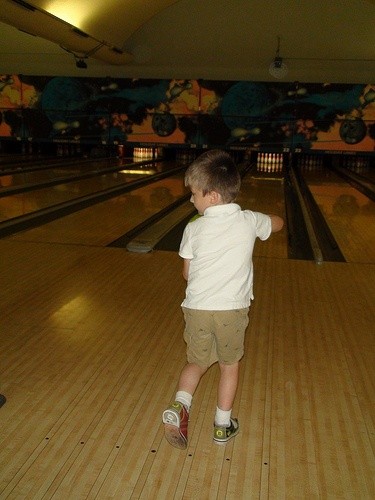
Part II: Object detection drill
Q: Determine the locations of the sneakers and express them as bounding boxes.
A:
[213,417,240,445]
[162,401,189,451]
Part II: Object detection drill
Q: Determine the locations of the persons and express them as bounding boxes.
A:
[162,150,283,452]
